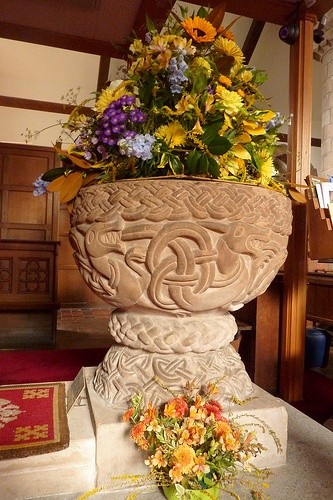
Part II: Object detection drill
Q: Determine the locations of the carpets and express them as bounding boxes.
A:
[0,382,70,460]
[1,347,110,384]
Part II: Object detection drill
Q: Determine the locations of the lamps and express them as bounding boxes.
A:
[313,15,328,44]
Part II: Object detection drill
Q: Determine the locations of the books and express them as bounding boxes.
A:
[304,175,332,229]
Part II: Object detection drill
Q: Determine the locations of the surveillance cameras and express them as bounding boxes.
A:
[313,28,324,44]
[279,22,299,44]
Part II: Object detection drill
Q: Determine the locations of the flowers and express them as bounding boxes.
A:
[19,2,311,206]
[75,374,283,499]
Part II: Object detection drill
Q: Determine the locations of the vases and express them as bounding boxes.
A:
[160,478,220,500]
[68,181,294,410]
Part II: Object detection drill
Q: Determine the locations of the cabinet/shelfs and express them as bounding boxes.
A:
[0,239,61,346]
